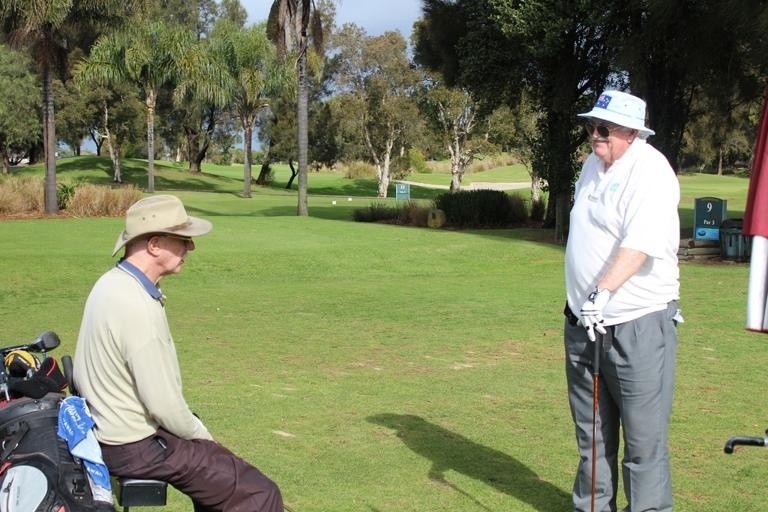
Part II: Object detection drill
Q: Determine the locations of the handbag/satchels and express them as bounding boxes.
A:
[564,300,578,325]
[0,395,117,512]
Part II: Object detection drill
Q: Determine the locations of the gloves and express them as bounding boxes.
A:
[577,284,612,341]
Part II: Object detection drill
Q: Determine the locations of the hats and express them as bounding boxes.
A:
[576,90,656,136]
[112,195,212,258]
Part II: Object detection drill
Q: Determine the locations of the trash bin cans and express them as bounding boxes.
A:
[720,218,751,261]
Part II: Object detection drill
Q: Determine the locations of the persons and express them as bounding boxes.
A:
[564,90,683,512]
[70,195,285,512]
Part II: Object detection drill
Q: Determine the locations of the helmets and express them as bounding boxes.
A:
[1,351,40,375]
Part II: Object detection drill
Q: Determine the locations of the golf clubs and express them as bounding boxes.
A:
[1,332,61,400]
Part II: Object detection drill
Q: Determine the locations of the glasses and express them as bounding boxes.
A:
[585,124,621,137]
